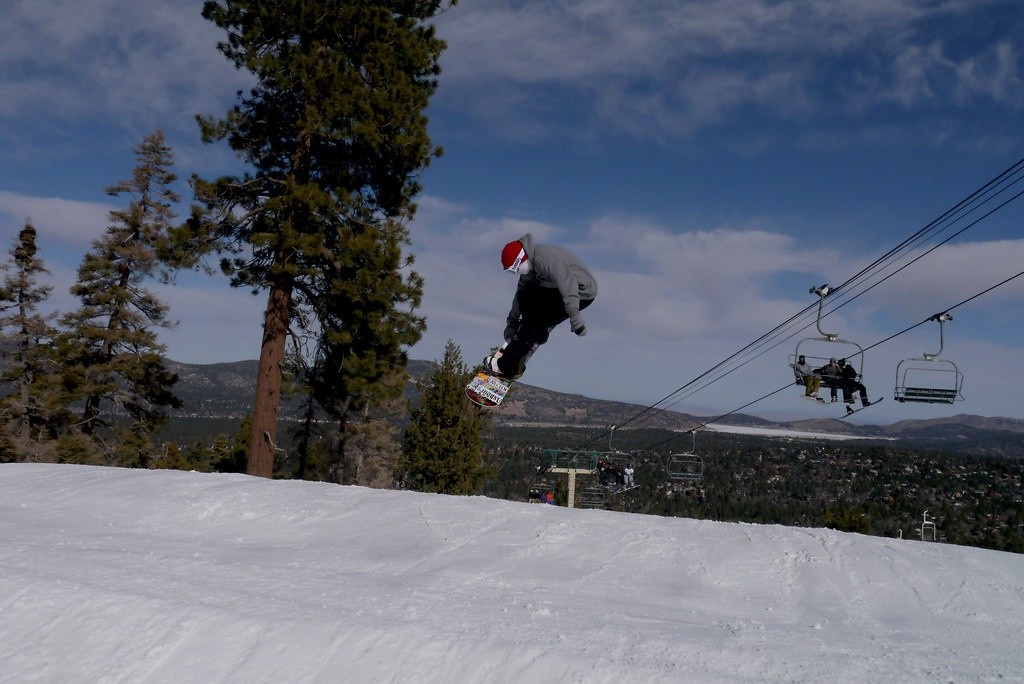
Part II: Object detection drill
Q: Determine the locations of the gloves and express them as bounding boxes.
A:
[570,312,587,336]
[503,325,516,343]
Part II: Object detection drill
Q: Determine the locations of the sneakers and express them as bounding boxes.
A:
[483,355,513,382]
[494,351,524,378]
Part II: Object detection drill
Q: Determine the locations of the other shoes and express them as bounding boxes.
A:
[832,397,837,402]
[862,400,869,406]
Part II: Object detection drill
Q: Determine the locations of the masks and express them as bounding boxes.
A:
[518,259,531,274]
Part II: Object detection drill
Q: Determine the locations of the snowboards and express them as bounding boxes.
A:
[464,323,558,408]
[613,484,628,495]
[839,396,884,419]
[620,484,641,492]
[799,394,860,405]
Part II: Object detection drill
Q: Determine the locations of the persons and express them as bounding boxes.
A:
[596,458,634,489]
[484,233,597,379]
[826,357,871,406]
[528,489,554,505]
[794,355,821,400]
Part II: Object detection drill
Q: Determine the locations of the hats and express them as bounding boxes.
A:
[501,240,528,270]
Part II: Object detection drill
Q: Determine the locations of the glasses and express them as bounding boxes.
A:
[507,258,522,274]
